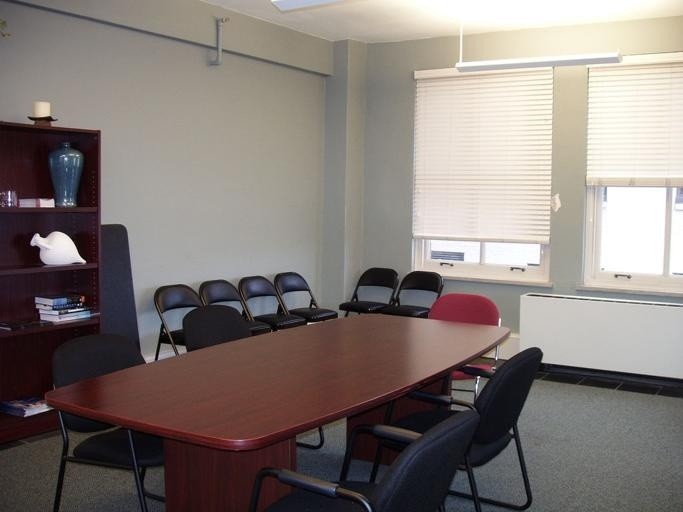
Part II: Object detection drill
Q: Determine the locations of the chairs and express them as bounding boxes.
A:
[429,292,502,408]
[183,305,251,353]
[238,276,306,332]
[152,284,203,359]
[52,331,166,512]
[369,346,543,511]
[199,279,271,336]
[247,409,478,510]
[338,267,399,318]
[274,272,338,325]
[381,271,444,318]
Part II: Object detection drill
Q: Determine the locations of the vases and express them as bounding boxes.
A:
[49,141,84,208]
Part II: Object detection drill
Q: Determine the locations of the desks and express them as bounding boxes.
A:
[43,316,512,510]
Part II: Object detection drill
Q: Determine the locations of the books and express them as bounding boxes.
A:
[15,196,56,209]
[0,394,55,418]
[0,293,101,332]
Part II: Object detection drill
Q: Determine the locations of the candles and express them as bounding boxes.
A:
[33,102,50,117]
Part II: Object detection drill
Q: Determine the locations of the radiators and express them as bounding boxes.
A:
[519,292,683,380]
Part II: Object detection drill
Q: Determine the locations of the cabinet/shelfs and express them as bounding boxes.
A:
[0,121,103,445]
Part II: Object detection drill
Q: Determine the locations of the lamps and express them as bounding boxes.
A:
[271,0,344,12]
[455,24,623,73]
